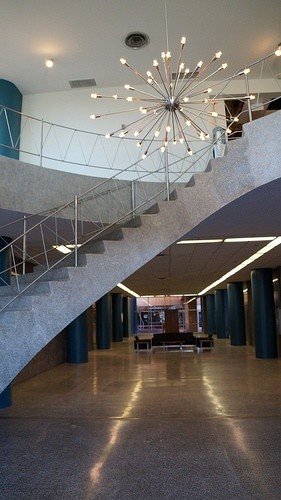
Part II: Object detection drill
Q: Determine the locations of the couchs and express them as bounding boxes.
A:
[152,332,197,352]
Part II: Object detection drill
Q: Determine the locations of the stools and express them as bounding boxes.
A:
[134,338,152,352]
[196,337,216,352]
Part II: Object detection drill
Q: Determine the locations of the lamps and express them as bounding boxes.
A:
[88,0,258,156]
